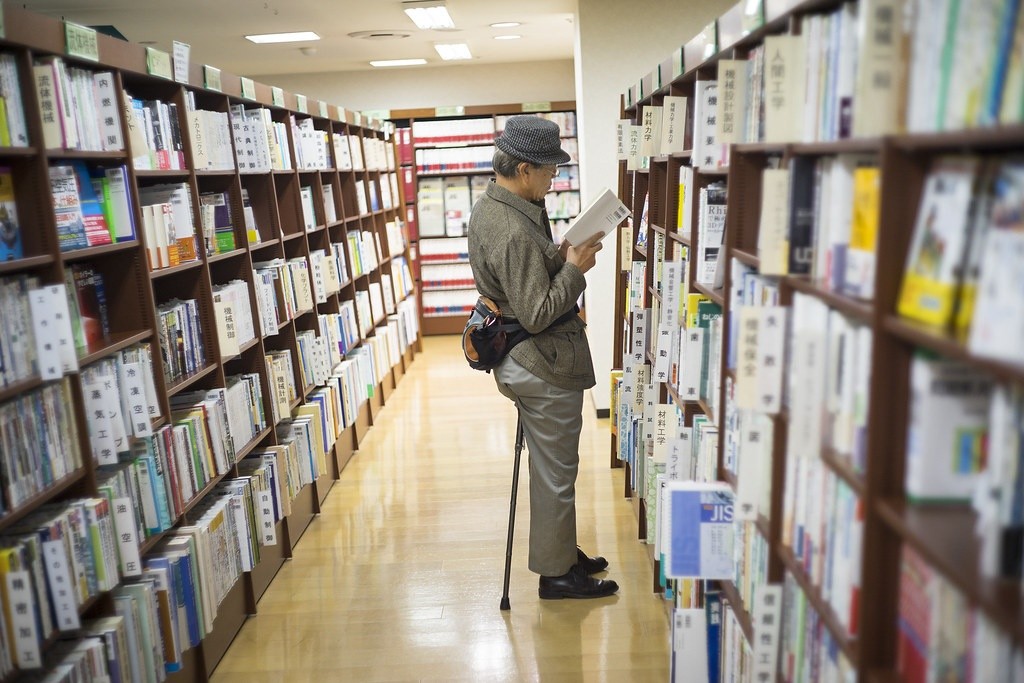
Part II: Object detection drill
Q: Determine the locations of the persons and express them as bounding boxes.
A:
[467,115,618,599]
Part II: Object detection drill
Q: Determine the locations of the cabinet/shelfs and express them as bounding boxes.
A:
[612,0,1024,683]
[0,5,421,683]
[388,100,581,336]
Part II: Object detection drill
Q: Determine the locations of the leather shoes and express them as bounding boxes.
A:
[539,565,619,599]
[577,545,608,573]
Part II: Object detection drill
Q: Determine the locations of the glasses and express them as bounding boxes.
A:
[518,161,560,178]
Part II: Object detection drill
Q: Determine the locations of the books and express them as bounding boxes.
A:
[0,0,1024,683]
[564,189,631,256]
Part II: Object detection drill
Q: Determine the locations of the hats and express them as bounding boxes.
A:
[495,116,571,165]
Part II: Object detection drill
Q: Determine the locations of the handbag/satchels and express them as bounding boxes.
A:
[462,296,506,373]
